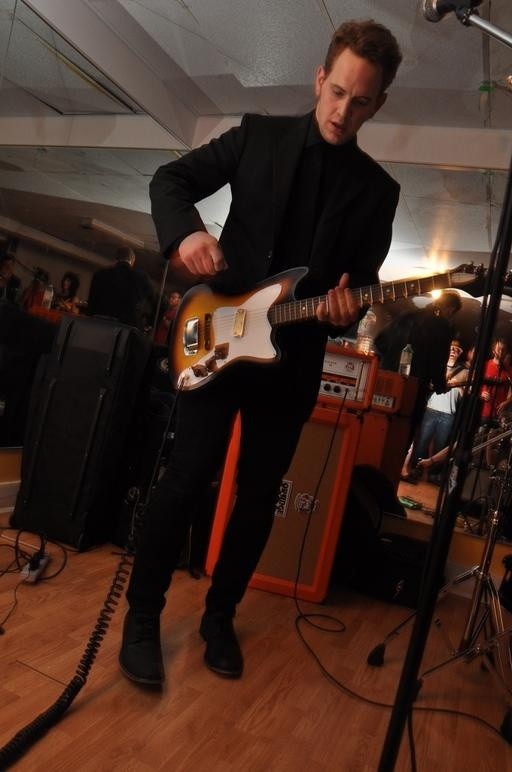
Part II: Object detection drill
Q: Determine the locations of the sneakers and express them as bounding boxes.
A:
[401,473,417,484]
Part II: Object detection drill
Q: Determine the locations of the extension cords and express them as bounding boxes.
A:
[21,550,51,581]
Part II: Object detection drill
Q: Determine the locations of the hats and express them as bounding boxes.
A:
[450,340,463,353]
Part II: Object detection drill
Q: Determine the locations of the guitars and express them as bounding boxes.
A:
[168,260,512,391]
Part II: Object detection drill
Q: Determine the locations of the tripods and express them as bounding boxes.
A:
[368,473,512,706]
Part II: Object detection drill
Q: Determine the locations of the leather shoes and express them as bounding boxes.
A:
[119,608,165,684]
[200,611,242,679]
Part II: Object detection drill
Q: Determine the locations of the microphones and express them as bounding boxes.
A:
[419,0,488,23]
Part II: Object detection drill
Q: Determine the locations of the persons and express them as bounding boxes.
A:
[374,291,511,484]
[0,246,181,347]
[116,20,402,687]
